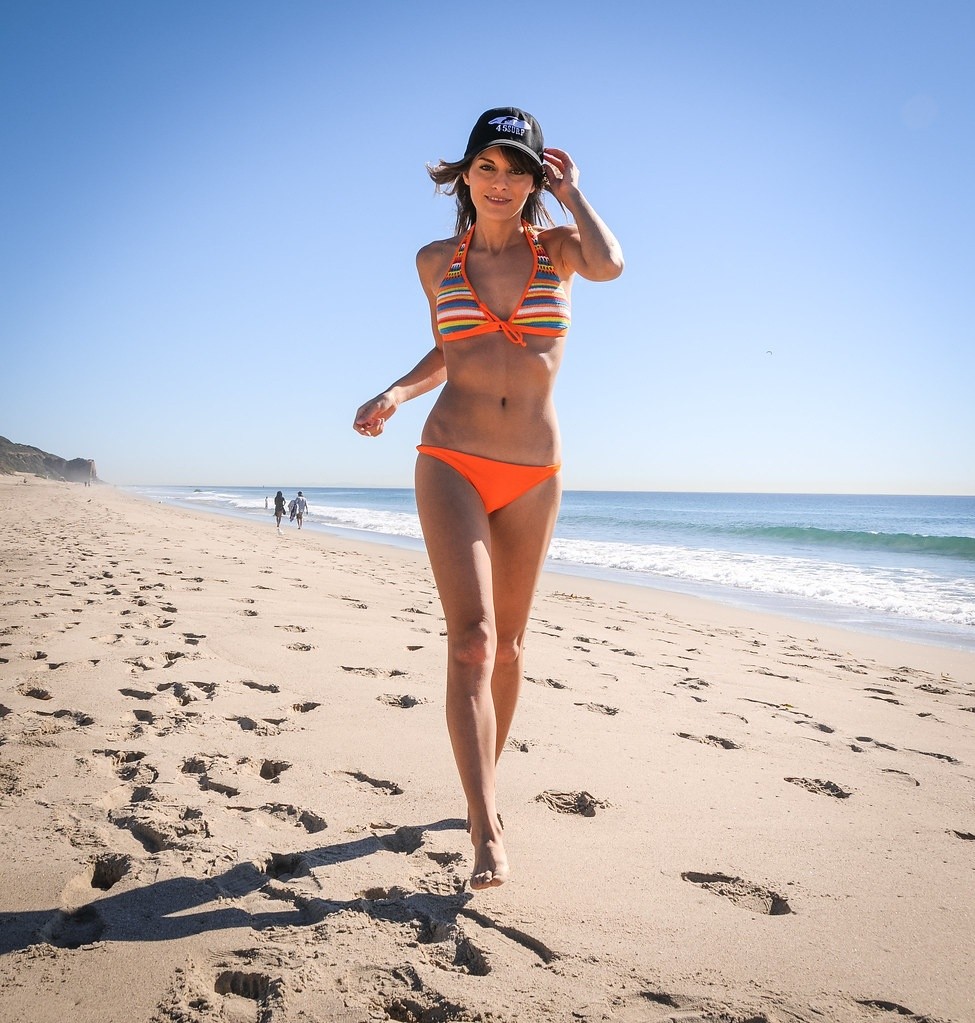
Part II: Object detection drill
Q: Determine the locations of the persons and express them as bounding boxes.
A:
[84,481,91,488]
[353,106,624,890]
[295,491,309,529]
[265,497,269,508]
[274,491,286,527]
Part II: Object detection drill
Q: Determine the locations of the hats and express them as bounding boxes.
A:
[464,107,544,167]
